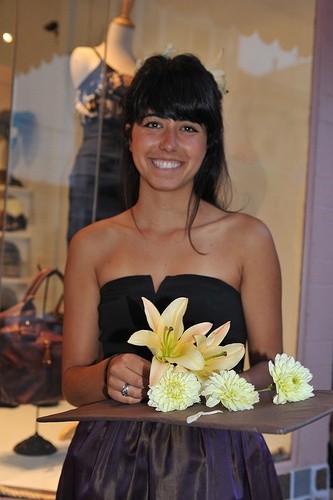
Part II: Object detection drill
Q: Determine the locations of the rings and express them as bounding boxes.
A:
[121,383,130,396]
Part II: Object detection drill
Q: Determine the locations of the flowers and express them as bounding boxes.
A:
[127,297,315,412]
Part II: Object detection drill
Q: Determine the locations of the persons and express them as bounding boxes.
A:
[66,0,156,272]
[55,51,283,500]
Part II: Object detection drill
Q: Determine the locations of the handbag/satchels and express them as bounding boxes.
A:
[0,267,64,404]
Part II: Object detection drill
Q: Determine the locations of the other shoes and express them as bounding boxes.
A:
[0,214,27,231]
[0,170,22,186]
